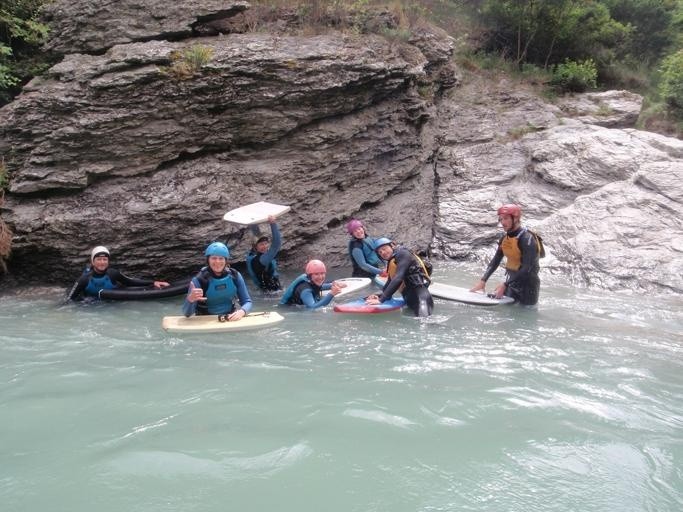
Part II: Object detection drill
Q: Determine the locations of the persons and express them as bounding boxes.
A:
[363,238,434,319]
[182,241,253,322]
[278,259,348,309]
[246,213,285,299]
[58,246,172,308]
[346,218,398,278]
[470,204,546,307]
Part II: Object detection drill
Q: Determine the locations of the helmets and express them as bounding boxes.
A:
[373,238,392,250]
[90,246,110,265]
[204,241,230,259]
[305,260,327,275]
[497,203,521,219]
[347,220,363,234]
[250,232,269,245]
